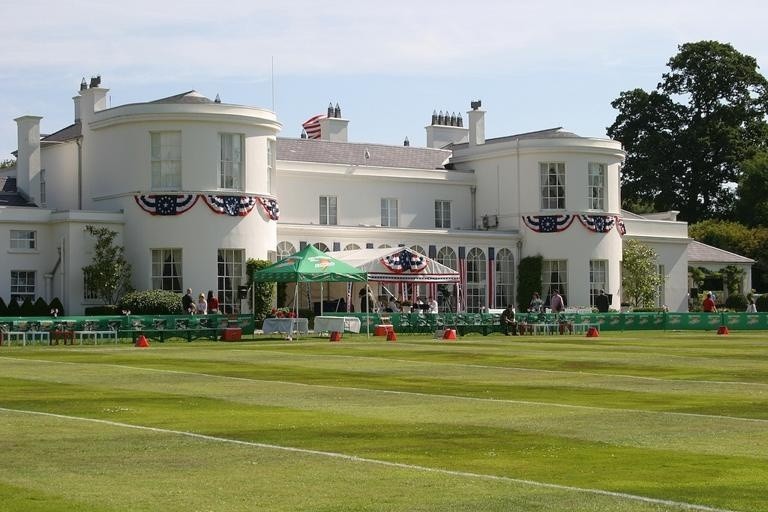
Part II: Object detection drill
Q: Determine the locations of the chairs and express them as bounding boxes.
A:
[398,310,502,336]
[1,317,217,347]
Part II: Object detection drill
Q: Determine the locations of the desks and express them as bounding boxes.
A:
[264,315,360,341]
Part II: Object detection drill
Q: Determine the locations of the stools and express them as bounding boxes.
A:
[518,323,601,336]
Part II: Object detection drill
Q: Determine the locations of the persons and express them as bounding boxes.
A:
[703,293,718,313]
[206,290,220,316]
[428,296,439,314]
[746,293,757,312]
[415,298,423,313]
[182,288,197,315]
[388,296,402,313]
[595,289,609,313]
[550,290,565,313]
[528,293,543,314]
[501,304,520,336]
[358,288,374,312]
[192,293,208,315]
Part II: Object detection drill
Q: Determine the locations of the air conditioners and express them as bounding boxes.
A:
[482,215,497,227]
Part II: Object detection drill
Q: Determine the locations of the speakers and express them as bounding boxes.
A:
[238,285,247,299]
[690,287,697,298]
